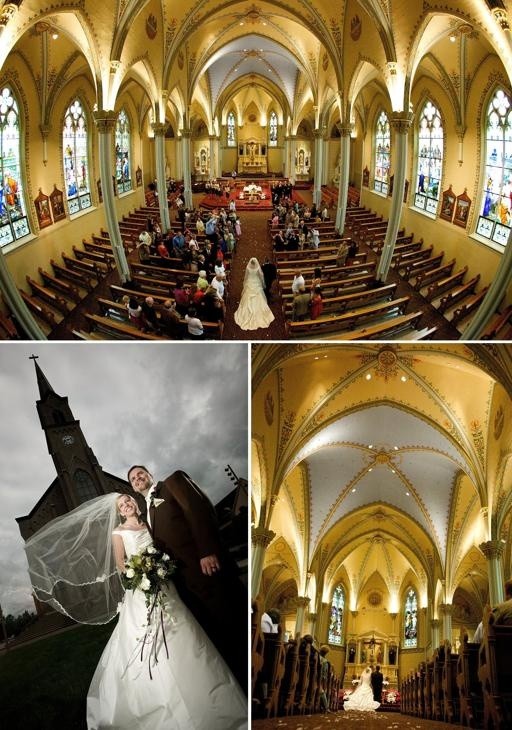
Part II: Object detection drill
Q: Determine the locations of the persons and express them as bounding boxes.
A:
[251,608,280,633]
[290,287,311,322]
[371,665,383,702]
[291,270,305,295]
[111,493,179,729]
[268,178,328,252]
[128,465,247,701]
[346,241,357,266]
[311,287,324,321]
[358,668,371,710]
[311,267,322,290]
[471,620,485,645]
[261,256,278,296]
[303,634,314,644]
[235,256,276,331]
[493,579,512,626]
[319,644,330,661]
[148,169,237,209]
[121,203,242,340]
[335,241,348,267]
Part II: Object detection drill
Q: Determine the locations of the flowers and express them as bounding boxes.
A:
[120,544,178,681]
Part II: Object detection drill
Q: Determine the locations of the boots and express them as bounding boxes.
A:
[320,692,331,714]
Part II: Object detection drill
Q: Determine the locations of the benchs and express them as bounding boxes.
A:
[251,593,342,719]
[269,184,512,340]
[0,180,231,340]
[400,603,512,730]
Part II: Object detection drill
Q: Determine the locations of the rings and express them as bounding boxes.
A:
[211,566,217,569]
